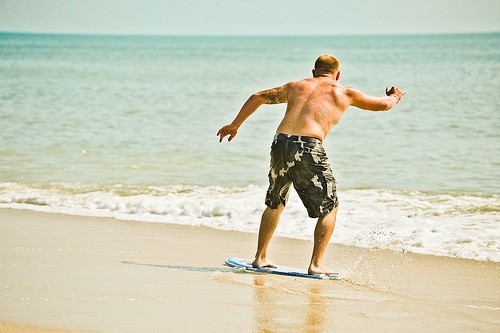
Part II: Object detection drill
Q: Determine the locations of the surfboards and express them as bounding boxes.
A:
[226,257,339,280]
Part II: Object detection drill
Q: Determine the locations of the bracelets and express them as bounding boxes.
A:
[387,93,400,104]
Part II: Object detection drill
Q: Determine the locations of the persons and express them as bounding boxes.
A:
[216,53,407,279]
